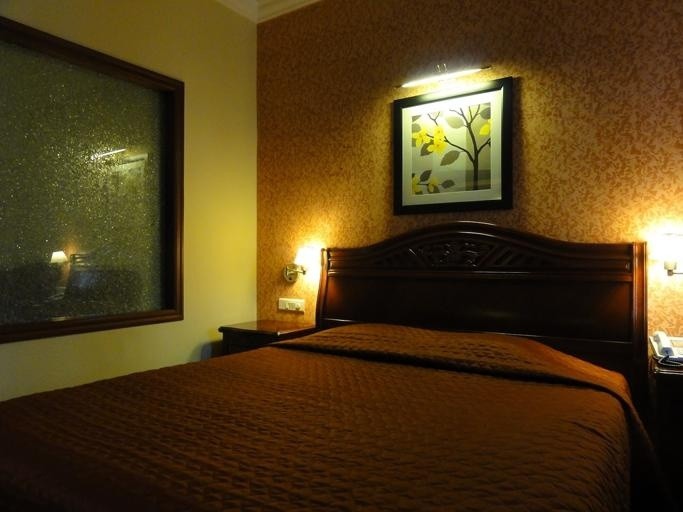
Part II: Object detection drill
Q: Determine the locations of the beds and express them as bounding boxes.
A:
[26,247,143,317]
[0,220,650,512]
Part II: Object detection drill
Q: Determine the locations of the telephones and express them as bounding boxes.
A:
[648,331,683,362]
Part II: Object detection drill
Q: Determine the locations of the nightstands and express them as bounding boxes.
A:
[217,318,315,355]
[648,352,681,483]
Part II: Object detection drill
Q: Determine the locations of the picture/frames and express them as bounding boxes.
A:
[389,75,514,216]
[107,152,152,199]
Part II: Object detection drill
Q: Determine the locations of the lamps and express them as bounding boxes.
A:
[281,243,323,284]
[48,250,72,266]
[393,65,493,91]
[657,228,682,280]
